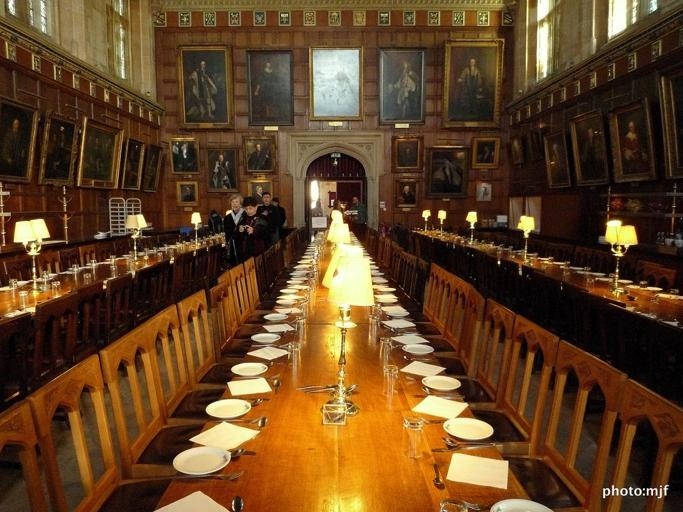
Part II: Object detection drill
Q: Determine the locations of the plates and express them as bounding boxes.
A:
[521,250,613,288]
[231,362,268,377]
[251,227,434,355]
[205,398,252,420]
[93,233,107,240]
[614,279,683,302]
[173,446,232,476]
[489,498,554,512]
[421,376,461,392]
[443,417,494,442]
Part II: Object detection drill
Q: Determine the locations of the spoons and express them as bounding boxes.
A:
[222,396,270,406]
[423,386,465,399]
[432,462,445,484]
[231,495,243,512]
[439,436,495,451]
[212,416,268,427]
[236,374,281,394]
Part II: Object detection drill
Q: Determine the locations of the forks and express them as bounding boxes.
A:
[164,471,244,479]
[466,501,492,511]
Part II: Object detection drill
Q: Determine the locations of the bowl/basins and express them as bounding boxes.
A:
[664,232,682,248]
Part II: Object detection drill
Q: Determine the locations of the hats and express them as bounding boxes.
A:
[272,197,279,203]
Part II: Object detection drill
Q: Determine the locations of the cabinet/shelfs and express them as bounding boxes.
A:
[108,195,141,237]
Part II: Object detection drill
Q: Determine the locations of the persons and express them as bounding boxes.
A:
[347,195,365,243]
[270,196,286,228]
[232,196,268,263]
[207,210,222,235]
[222,194,246,265]
[328,198,346,224]
[0,56,658,205]
[255,192,280,228]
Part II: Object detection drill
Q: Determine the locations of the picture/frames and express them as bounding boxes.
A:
[0,91,163,194]
[390,135,502,214]
[542,60,682,191]
[166,136,277,208]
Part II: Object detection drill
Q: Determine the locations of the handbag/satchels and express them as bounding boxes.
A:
[227,240,237,264]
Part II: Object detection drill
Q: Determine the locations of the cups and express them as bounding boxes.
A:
[122,241,186,262]
[186,231,226,248]
[436,229,513,258]
[440,500,468,512]
[384,363,400,396]
[401,416,425,459]
[9,255,118,310]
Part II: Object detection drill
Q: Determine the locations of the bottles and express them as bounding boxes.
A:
[611,197,671,213]
[481,218,497,228]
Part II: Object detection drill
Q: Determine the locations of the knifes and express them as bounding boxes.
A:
[227,448,256,458]
[297,380,359,393]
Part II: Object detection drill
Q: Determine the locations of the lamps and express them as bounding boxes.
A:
[516,216,535,264]
[436,210,446,238]
[465,211,477,245]
[603,218,637,296]
[320,210,373,417]
[124,213,148,266]
[11,218,50,295]
[190,211,202,250]
[421,210,431,233]
